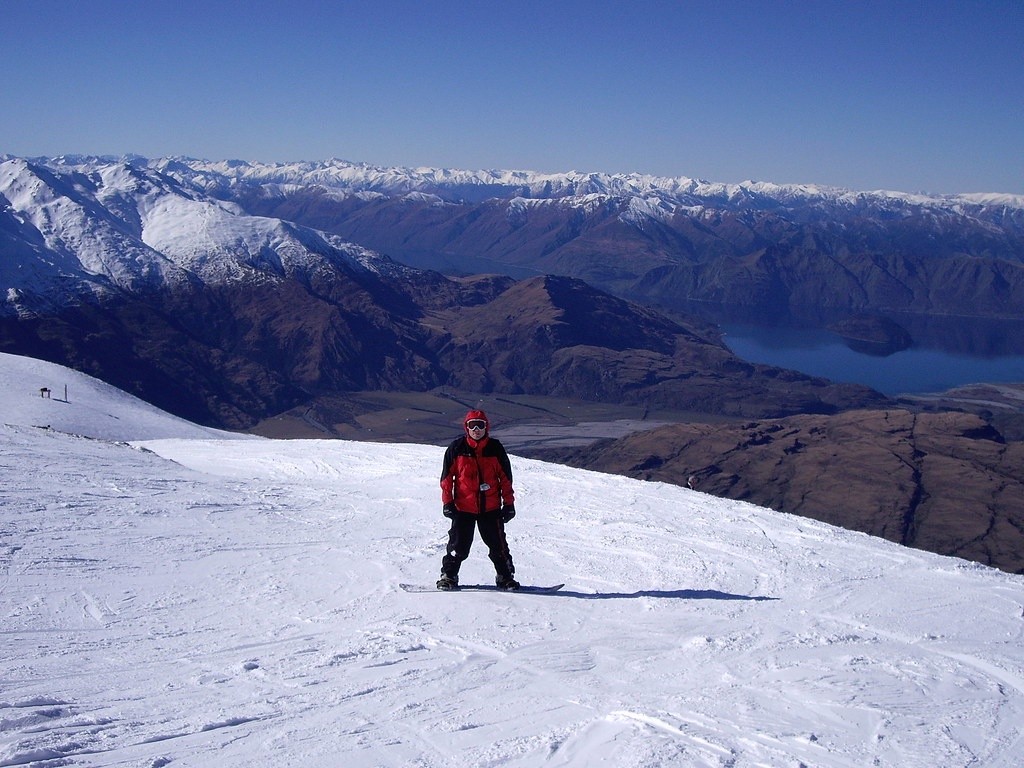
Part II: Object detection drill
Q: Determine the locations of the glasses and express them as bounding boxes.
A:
[467,419,486,431]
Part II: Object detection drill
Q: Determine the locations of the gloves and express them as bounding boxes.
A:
[502,504,515,523]
[443,503,458,519]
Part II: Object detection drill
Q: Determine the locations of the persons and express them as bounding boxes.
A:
[440,409,521,591]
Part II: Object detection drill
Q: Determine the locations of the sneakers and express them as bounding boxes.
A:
[436,573,458,590]
[496,575,520,590]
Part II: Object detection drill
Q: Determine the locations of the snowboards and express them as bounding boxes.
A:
[400,581,565,595]
